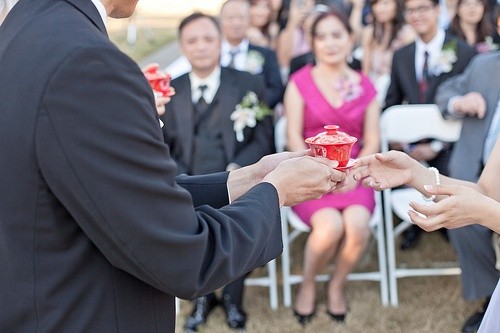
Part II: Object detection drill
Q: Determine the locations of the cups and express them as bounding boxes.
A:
[140,64,171,101]
[304,125,357,172]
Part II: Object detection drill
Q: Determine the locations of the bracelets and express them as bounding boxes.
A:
[420,165,443,202]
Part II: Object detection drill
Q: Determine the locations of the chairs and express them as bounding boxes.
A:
[273,114,389,308]
[379,104,500,309]
[173,101,287,316]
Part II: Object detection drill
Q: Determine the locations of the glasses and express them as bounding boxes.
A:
[403,5,435,16]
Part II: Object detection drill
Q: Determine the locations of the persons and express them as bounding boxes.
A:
[344,129,500,332]
[199,0,500,106]
[0,0,349,332]
[378,0,481,253]
[139,63,171,115]
[431,0,500,331]
[280,7,383,325]
[148,10,280,330]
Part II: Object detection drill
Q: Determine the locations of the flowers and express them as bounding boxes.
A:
[229,89,274,143]
[428,39,458,75]
[334,70,364,105]
[475,36,498,55]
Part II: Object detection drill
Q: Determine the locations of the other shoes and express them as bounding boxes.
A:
[463,309,484,333]
[293,310,315,326]
[326,309,348,323]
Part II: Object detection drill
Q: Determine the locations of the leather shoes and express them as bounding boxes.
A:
[182,297,218,329]
[220,303,248,329]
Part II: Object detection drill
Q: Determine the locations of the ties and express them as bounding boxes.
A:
[226,49,239,69]
[194,85,210,126]
[423,50,431,90]
